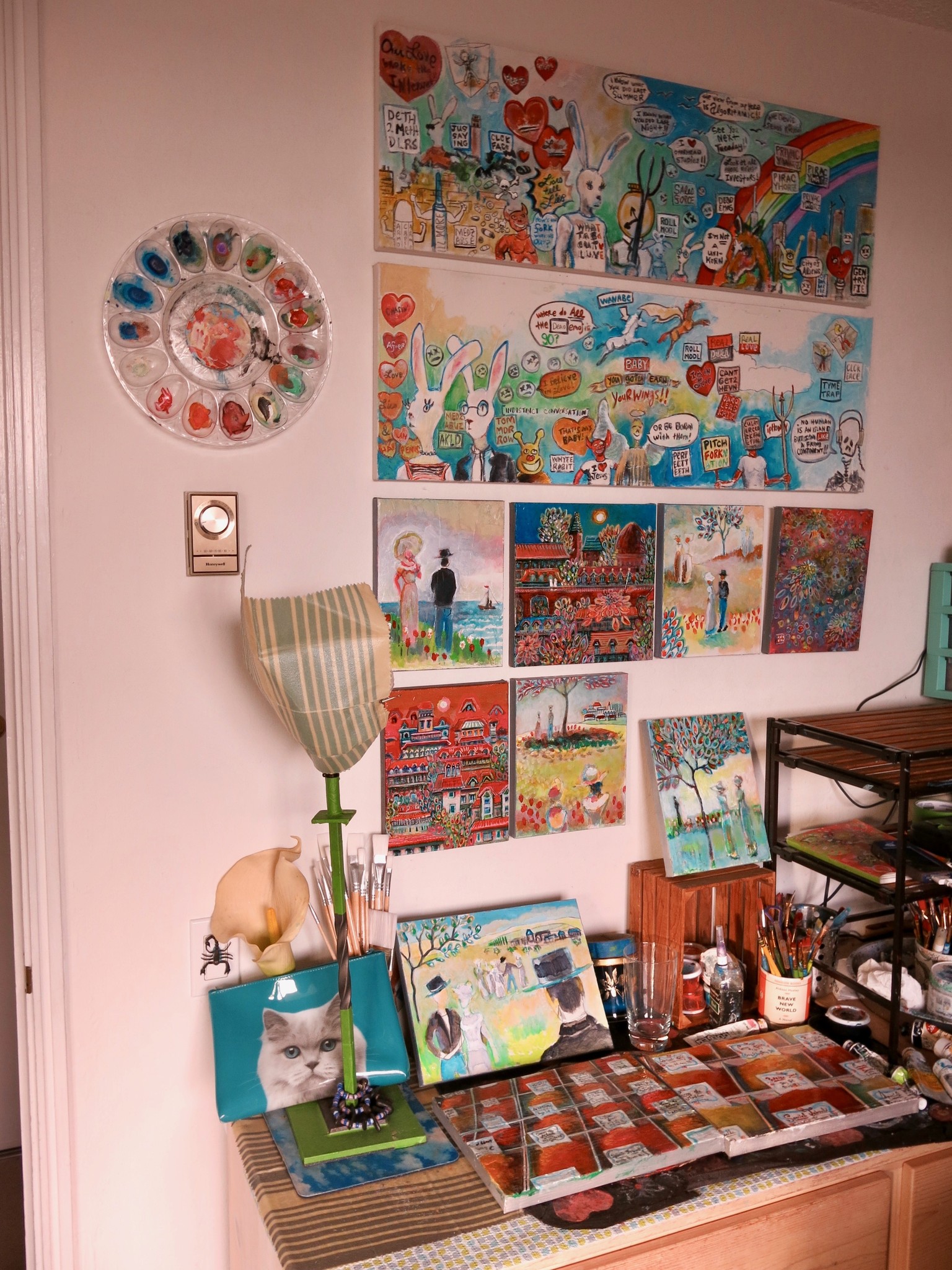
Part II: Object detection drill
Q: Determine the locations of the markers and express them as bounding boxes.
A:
[942,943,950,955]
[932,927,947,954]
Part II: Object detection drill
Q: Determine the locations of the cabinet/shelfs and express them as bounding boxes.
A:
[762,695,952,1084]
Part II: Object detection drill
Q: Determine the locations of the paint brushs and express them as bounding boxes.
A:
[372,834,389,911]
[907,897,952,956]
[755,890,851,978]
[313,832,369,957]
[309,902,337,963]
[358,848,366,956]
[384,855,393,912]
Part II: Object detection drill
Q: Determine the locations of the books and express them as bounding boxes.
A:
[786,818,952,884]
[431,1052,725,1215]
[638,1024,919,1158]
[394,898,614,1087]
[640,712,772,877]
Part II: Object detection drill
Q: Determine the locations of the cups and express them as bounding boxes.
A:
[914,938,951,1023]
[758,965,813,1031]
[622,941,678,1052]
[682,943,711,1015]
[584,933,638,1023]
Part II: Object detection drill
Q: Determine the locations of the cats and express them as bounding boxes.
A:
[256,991,372,1114]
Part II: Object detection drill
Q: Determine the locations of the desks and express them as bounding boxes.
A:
[215,1043,952,1270]
